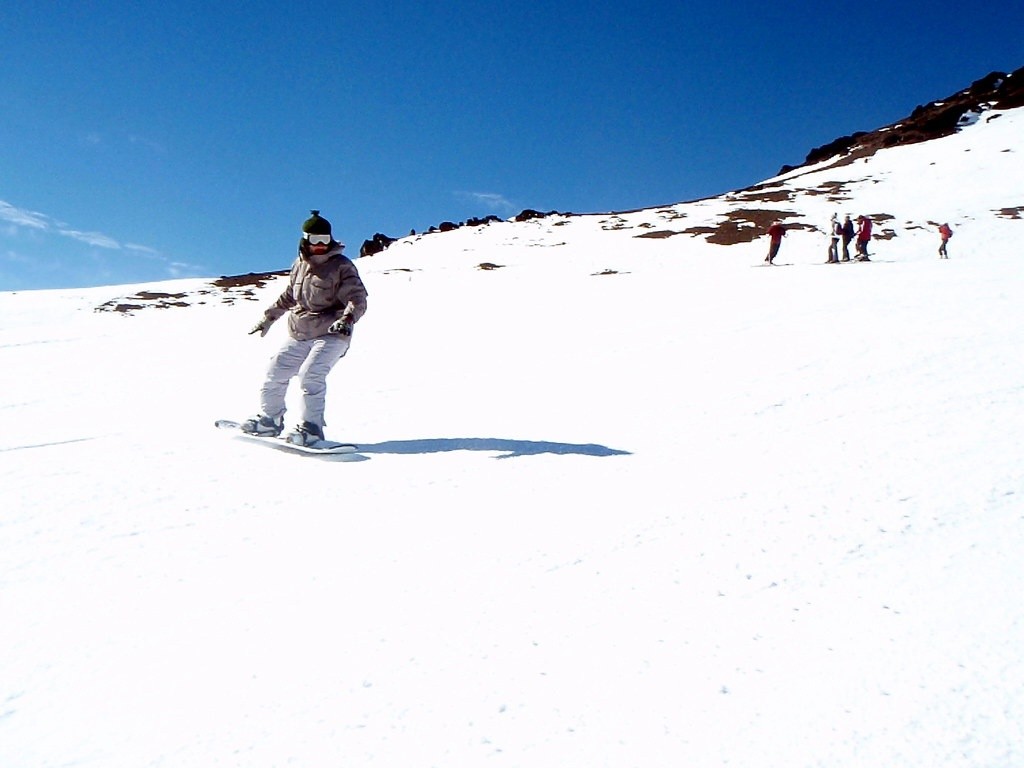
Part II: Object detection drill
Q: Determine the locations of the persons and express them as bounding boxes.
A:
[242,209,369,447]
[937,223,953,259]
[764,220,788,265]
[827,212,872,262]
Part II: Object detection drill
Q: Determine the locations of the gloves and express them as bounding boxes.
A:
[328,316,353,336]
[248,316,272,336]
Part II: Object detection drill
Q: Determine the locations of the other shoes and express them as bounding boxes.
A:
[243,414,284,436]
[287,425,324,447]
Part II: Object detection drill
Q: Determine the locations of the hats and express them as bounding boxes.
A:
[300,210,332,248]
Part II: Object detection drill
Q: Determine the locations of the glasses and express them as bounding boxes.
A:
[309,234,330,245]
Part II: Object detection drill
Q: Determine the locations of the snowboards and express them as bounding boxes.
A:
[216,420,359,453]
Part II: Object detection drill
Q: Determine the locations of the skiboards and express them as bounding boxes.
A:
[751,263,795,268]
[814,252,877,266]
